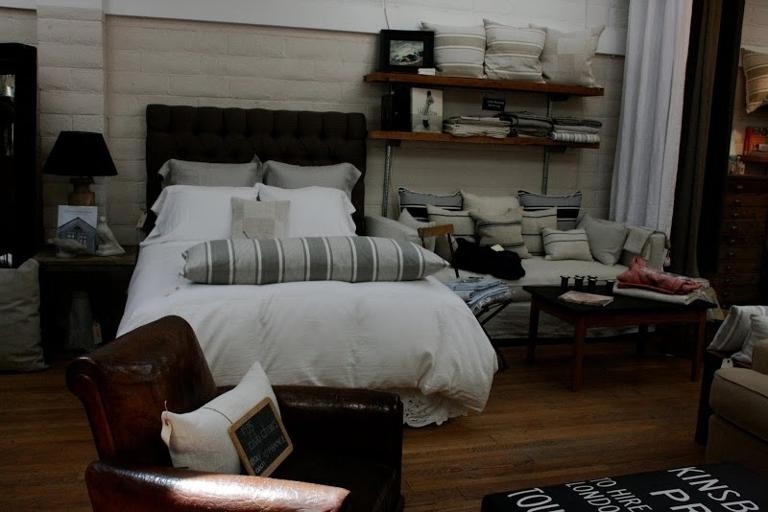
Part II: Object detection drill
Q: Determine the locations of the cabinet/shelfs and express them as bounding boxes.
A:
[357,67,606,155]
[0,39,44,271]
[702,150,767,340]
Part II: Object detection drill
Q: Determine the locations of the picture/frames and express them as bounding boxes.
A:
[375,27,437,75]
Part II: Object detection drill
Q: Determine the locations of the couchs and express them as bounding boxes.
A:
[360,211,672,347]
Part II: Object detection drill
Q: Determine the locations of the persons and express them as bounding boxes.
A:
[730,154,745,176]
[420,90,435,131]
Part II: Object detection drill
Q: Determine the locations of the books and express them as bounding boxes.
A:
[558,290,615,307]
[55,204,98,257]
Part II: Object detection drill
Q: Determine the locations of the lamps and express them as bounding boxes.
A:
[38,126,120,209]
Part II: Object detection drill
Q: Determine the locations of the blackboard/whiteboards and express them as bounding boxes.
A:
[227,397,294,478]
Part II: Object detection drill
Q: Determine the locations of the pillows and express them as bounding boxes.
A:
[261,160,362,192]
[179,237,451,284]
[704,302,767,359]
[230,194,291,238]
[416,15,610,92]
[727,312,767,364]
[0,257,52,375]
[740,44,768,115]
[147,185,257,240]
[388,178,634,268]
[258,182,356,238]
[159,156,260,187]
[156,357,287,480]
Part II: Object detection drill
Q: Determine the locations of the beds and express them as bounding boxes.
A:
[98,98,506,437]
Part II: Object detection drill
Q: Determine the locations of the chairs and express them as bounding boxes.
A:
[64,310,409,512]
[693,303,767,455]
[412,218,517,379]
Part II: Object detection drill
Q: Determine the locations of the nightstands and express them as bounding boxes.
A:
[29,237,139,373]
